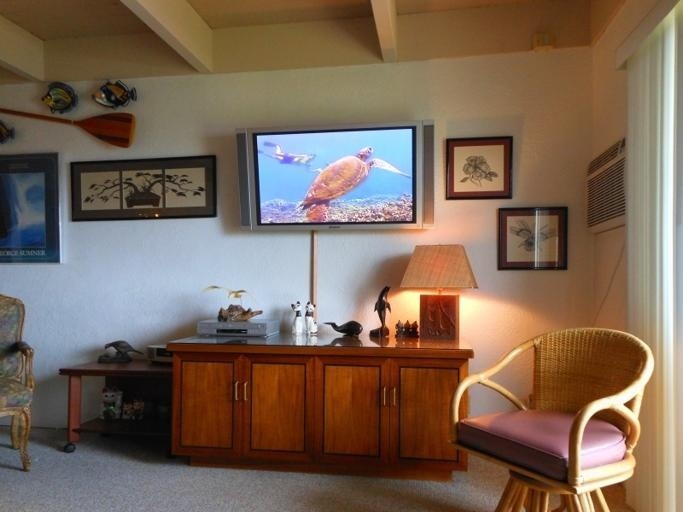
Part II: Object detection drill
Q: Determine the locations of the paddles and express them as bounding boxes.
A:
[0,108,134,147]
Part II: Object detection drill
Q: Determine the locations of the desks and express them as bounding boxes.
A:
[59,358,172,442]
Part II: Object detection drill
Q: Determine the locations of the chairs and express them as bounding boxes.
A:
[448,326,655,512]
[0,292,36,472]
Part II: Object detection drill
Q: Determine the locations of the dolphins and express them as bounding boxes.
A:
[374,286,392,329]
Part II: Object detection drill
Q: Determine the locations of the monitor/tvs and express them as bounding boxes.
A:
[246,119,424,231]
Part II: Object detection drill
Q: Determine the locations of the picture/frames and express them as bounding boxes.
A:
[444,135,514,200]
[0,152,61,264]
[70,155,217,222]
[498,205,569,270]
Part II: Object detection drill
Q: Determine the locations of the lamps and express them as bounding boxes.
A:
[399,244,479,341]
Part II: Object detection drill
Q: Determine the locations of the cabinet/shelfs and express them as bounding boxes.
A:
[315,356,468,471]
[172,352,315,464]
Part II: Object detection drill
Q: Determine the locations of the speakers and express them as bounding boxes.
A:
[235,127,252,232]
[422,119,437,229]
